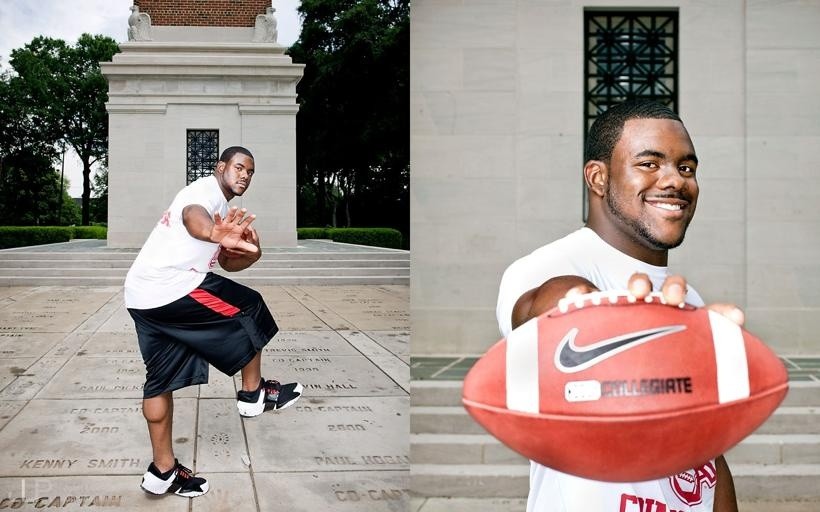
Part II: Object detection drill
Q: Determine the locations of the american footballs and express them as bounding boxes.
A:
[221,227,258,258]
[460,290,790,482]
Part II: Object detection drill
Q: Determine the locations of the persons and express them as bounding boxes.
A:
[495,91,746,512]
[122,145,304,499]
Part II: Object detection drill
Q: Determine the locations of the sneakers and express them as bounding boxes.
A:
[139,453,210,500]
[236,378,303,418]
